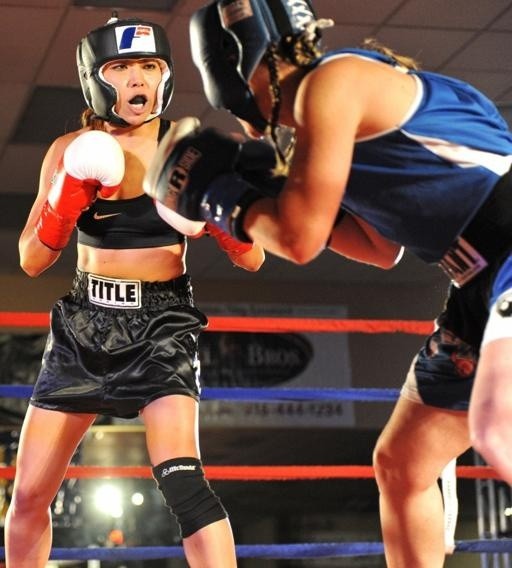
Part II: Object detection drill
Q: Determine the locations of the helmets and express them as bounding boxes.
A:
[75,21,174,127]
[188,0,323,114]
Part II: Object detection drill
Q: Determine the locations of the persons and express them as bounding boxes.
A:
[142,1,510,568]
[0,16,240,568]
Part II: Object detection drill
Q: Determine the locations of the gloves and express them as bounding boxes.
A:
[33,131,125,252]
[142,117,259,241]
